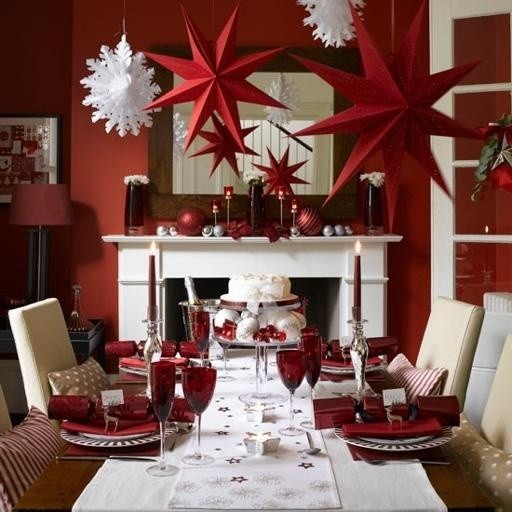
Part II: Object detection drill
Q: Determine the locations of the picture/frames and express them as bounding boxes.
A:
[0,114,63,207]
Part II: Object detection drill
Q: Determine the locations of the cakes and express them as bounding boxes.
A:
[212,274,307,342]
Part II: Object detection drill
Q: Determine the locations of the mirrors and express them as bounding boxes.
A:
[146,47,358,220]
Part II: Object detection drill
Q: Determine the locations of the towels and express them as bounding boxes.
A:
[321,357,383,367]
[440,414,512,511]
[121,357,190,369]
[343,418,444,437]
[60,419,159,435]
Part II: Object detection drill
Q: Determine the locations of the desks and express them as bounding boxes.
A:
[0,319,105,426]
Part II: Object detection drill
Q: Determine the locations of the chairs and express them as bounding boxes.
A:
[0,385,12,431]
[481,334,512,452]
[9,298,79,416]
[415,296,484,412]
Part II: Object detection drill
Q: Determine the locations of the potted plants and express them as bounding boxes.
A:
[124,175,150,185]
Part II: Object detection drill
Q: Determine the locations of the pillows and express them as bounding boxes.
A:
[387,354,448,405]
[0,406,65,512]
[48,357,109,404]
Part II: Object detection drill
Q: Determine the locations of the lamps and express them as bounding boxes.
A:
[10,184,73,303]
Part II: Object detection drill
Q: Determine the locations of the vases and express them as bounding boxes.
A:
[249,187,266,236]
[365,183,383,236]
[125,181,144,236]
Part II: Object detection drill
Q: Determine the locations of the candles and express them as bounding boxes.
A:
[147,256,157,320]
[353,254,361,321]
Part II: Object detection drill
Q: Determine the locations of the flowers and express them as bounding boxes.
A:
[242,172,267,186]
[360,172,385,188]
